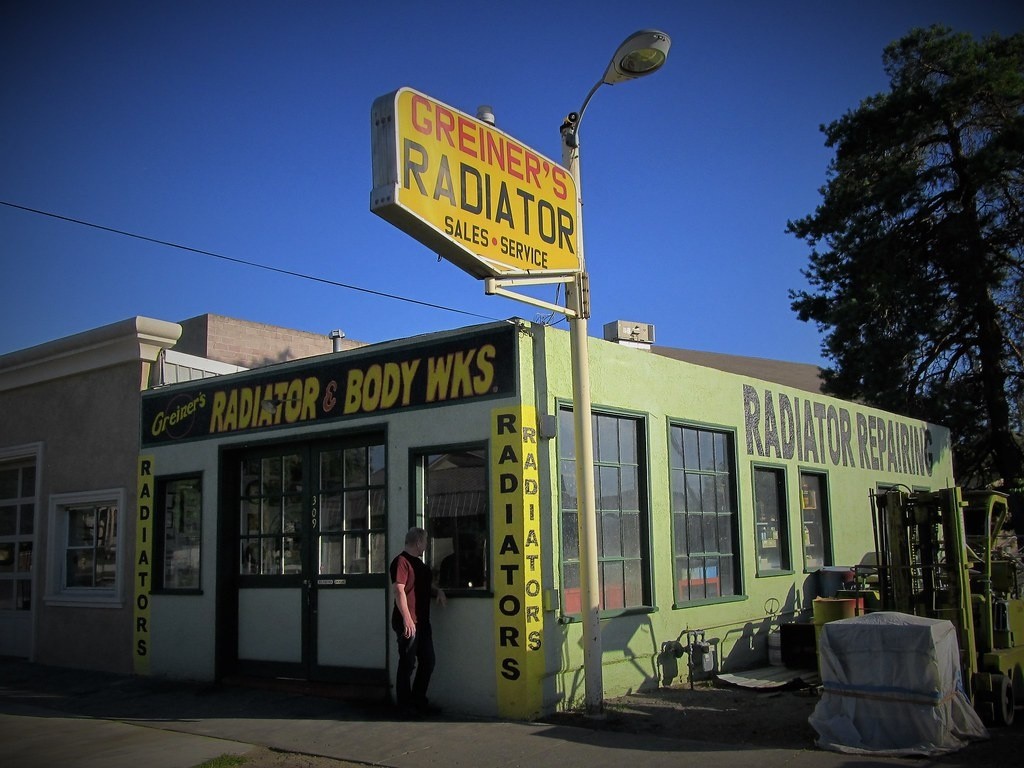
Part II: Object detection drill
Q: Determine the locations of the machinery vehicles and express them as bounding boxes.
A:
[849,481,1024,729]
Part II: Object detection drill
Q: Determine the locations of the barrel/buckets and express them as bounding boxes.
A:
[819,565,853,600]
[768,634,786,666]
[779,623,817,671]
[812,596,866,681]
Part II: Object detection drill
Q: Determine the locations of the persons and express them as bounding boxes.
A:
[389,527,447,713]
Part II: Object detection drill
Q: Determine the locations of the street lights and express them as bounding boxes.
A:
[562,27,674,727]
